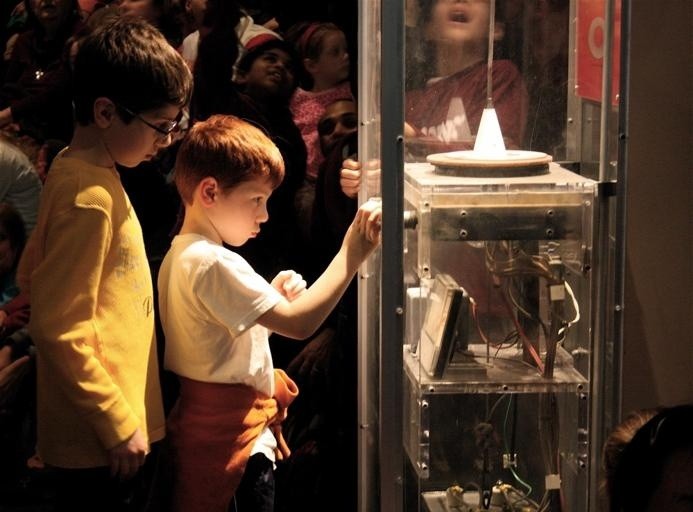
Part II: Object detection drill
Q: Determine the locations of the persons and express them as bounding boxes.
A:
[594,397,693,510]
[157,114,383,512]
[15,5,195,510]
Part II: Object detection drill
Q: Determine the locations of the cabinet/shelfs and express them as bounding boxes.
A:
[402,155,597,512]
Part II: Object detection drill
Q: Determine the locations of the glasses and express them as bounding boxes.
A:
[115,99,184,137]
